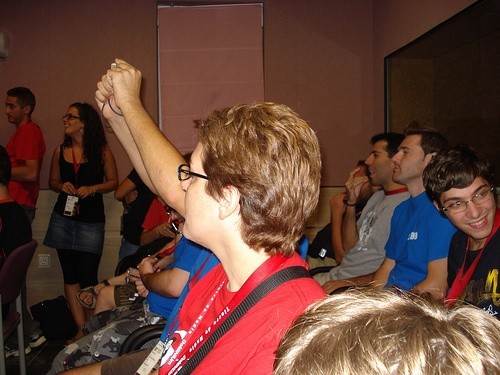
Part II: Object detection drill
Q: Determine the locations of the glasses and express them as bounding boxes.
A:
[178,164,209,180]
[62,113,79,120]
[439,182,495,214]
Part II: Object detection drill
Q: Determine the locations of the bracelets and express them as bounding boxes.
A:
[136,285,147,294]
[343,198,356,206]
[89,185,95,197]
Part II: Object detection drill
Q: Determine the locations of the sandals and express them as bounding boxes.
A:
[76,279,111,309]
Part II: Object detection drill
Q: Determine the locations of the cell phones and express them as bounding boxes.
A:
[172,223,178,232]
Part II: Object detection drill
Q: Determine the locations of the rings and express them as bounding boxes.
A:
[66,185,70,188]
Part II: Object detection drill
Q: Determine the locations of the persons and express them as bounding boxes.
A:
[0,145,32,269]
[370,120,458,299]
[423,144,500,321]
[4,88,46,357]
[272,283,500,375]
[312,133,411,294]
[47,58,385,375]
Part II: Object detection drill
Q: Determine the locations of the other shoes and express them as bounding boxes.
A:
[5,344,31,358]
[28,333,46,347]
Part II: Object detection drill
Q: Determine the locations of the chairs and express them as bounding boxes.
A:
[0,238,38,375]
